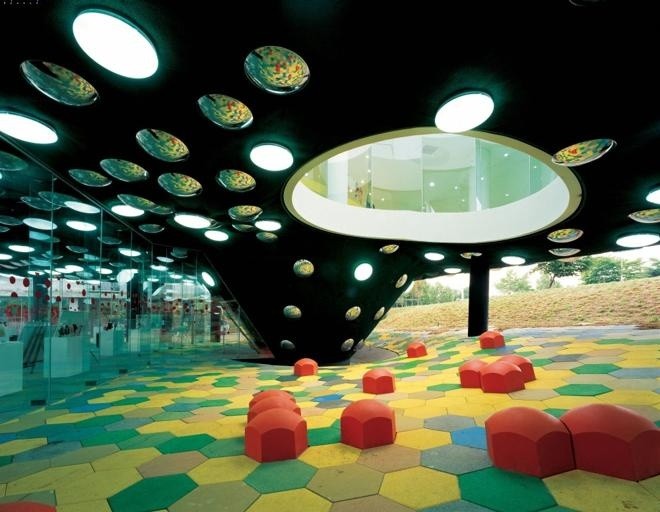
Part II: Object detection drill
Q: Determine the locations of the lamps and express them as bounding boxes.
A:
[432,86,495,135]
[248,141,294,174]
[1,107,60,146]
[72,8,159,81]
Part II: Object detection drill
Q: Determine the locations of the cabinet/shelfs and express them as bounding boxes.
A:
[1,322,160,399]
